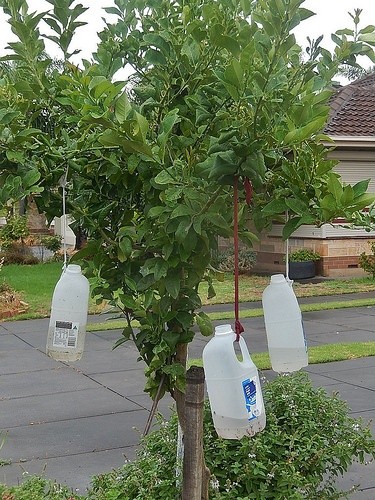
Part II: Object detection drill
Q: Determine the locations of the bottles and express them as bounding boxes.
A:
[201,323,267,440]
[259,270,309,373]
[44,261,93,367]
